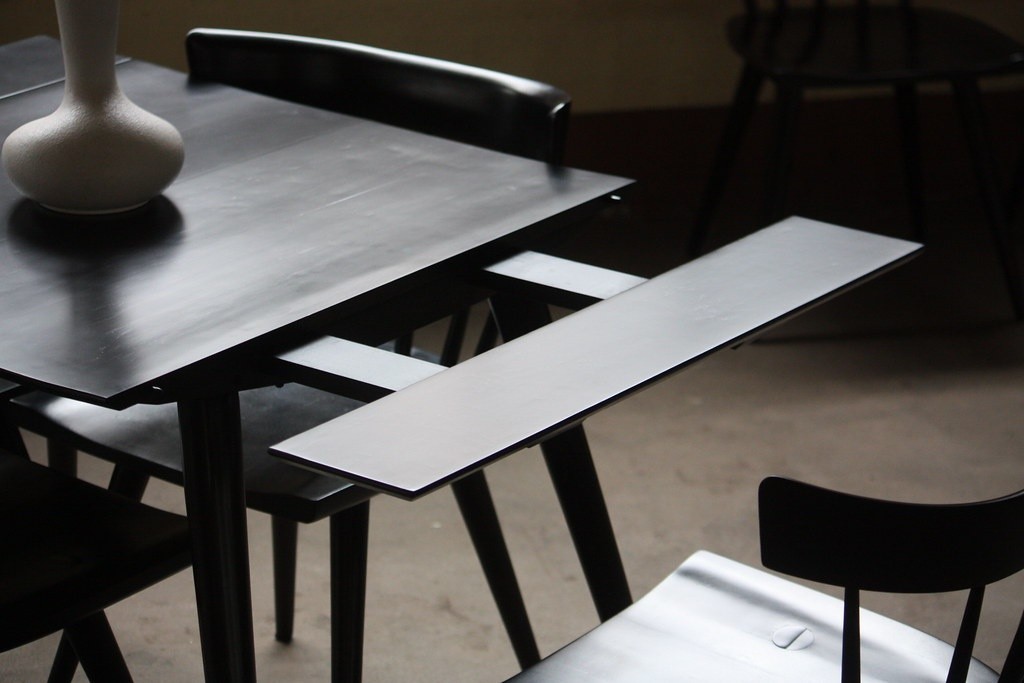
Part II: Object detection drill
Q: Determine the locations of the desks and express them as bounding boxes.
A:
[0,36,928,683]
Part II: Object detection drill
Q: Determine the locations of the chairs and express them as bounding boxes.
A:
[502,473,1024,683]
[11,28,575,683]
[682,0,1024,319]
[0,450,190,683]
